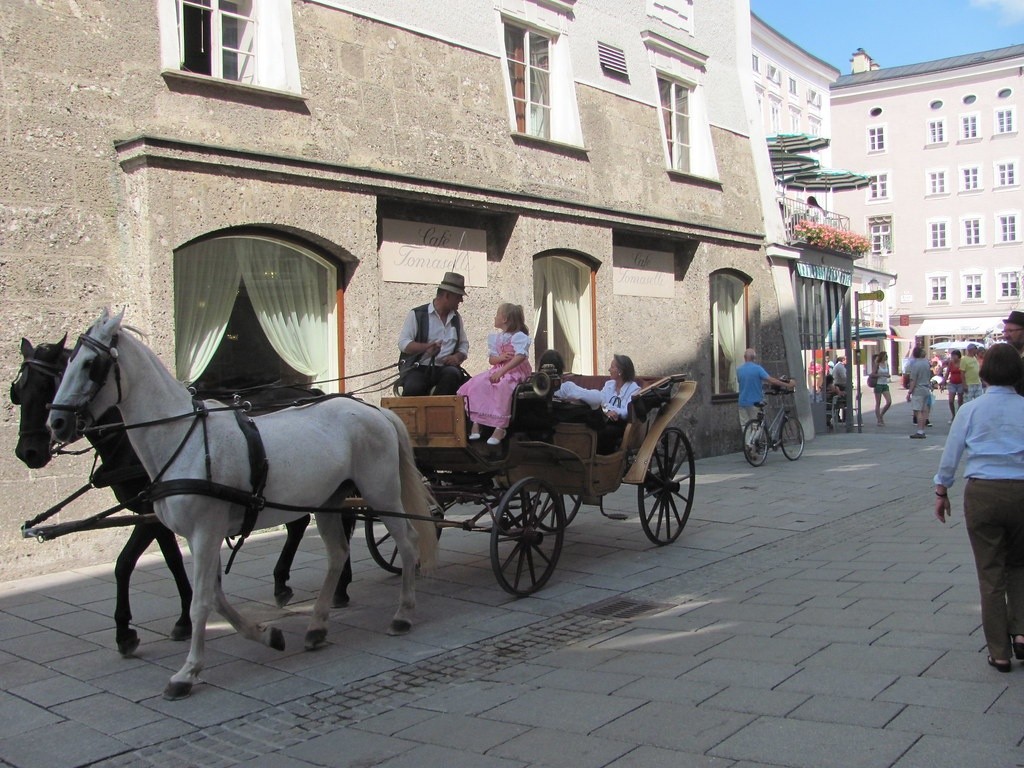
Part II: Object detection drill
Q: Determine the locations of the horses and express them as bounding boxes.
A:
[15,331,356,657]
[47,305,439,700]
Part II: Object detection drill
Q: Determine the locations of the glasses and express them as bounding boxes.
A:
[1002,329,1024,333]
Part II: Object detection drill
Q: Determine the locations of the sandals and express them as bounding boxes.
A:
[1014,634,1024,659]
[988,656,1011,672]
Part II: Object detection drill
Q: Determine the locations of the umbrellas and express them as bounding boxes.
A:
[770,154,820,180]
[781,169,873,213]
[768,133,831,192]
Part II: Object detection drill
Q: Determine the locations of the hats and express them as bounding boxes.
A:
[438,272,469,297]
[1003,311,1024,324]
[967,343,979,350]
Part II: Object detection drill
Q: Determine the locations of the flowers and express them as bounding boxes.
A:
[794,219,873,257]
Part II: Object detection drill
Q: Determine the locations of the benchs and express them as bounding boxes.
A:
[535,373,665,420]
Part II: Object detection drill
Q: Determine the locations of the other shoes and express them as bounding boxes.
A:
[912,416,918,425]
[910,433,926,438]
[948,416,955,423]
[469,433,480,440]
[487,428,507,445]
[925,418,933,427]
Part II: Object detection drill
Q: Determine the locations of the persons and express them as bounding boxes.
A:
[929,344,985,422]
[871,352,892,427]
[397,272,468,396]
[737,349,796,457]
[807,197,824,224]
[933,343,1024,672]
[540,349,601,409]
[598,355,641,454]
[457,303,532,445]
[1002,311,1024,362]
[808,355,846,427]
[907,347,933,439]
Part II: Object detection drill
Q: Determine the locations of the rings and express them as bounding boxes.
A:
[608,416,610,418]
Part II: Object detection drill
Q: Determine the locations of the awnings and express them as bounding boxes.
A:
[916,316,1008,336]
[851,327,887,338]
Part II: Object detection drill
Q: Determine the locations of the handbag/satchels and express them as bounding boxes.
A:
[867,377,877,387]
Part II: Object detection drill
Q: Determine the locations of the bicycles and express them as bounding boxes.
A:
[742,374,805,467]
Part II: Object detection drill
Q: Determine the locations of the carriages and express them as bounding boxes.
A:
[10,304,698,701]
